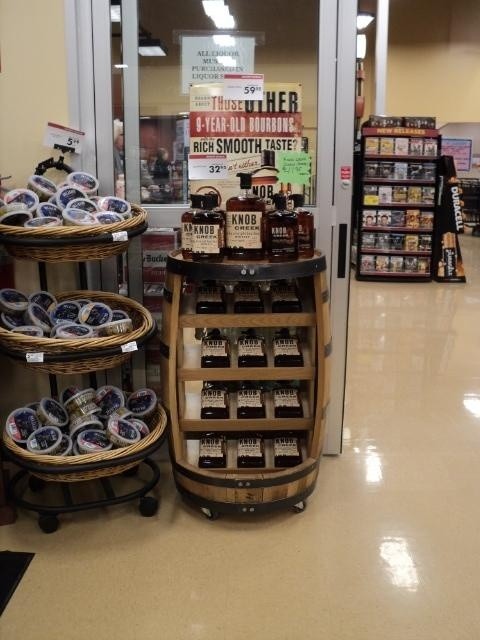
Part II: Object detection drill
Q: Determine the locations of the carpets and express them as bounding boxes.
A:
[0,550,37,621]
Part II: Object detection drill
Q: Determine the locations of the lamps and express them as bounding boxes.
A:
[110,28,170,61]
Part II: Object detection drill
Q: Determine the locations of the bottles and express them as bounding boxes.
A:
[198,431,304,468]
[225,170,265,261]
[249,148,285,213]
[115,174,125,200]
[261,192,299,262]
[286,193,316,260]
[195,279,304,313]
[200,380,305,418]
[200,328,305,367]
[179,191,228,263]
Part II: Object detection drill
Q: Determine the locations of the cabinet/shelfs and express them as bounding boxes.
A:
[0,199,335,535]
[353,122,443,286]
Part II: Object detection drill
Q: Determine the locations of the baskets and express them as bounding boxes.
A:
[1,384,166,484]
[0,195,147,264]
[0,290,155,376]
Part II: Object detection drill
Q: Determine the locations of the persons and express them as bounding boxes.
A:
[365,215,375,225]
[379,215,391,226]
[113,118,125,185]
[152,145,172,178]
